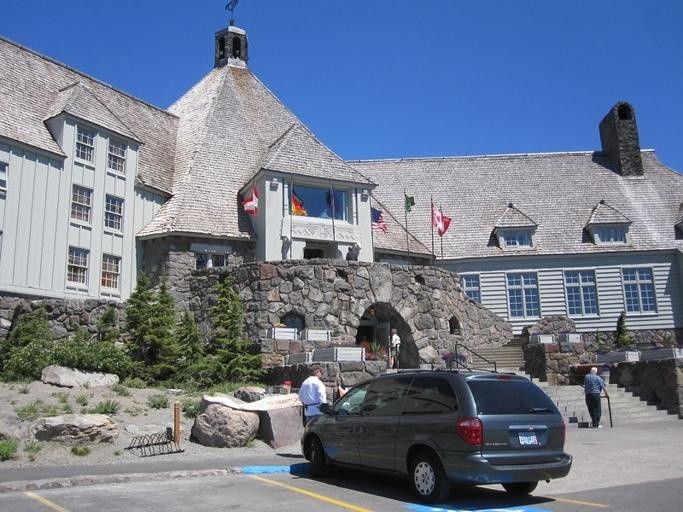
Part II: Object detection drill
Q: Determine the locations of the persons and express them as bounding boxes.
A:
[405,195,415,213]
[290,189,306,216]
[241,188,260,215]
[437,213,452,236]
[298,366,329,423]
[583,367,609,428]
[345,246,359,262]
[371,207,388,234]
[390,329,401,368]
[431,201,444,231]
[327,186,337,209]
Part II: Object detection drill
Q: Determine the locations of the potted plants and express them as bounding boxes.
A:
[368,339,381,360]
[442,352,466,369]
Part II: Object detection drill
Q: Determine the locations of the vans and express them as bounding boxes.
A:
[298,369,574,507]
